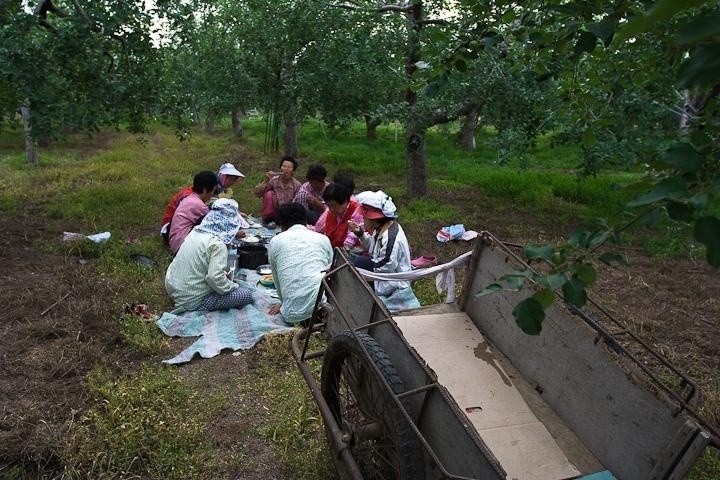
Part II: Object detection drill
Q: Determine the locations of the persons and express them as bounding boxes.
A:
[169,171,218,256]
[205,162,245,203]
[160,178,220,243]
[255,156,411,329]
[164,198,255,312]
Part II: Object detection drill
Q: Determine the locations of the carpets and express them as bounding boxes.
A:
[154,213,422,366]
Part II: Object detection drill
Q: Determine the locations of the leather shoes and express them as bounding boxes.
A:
[362,204,386,220]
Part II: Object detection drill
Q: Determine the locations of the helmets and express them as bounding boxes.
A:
[212,198,251,229]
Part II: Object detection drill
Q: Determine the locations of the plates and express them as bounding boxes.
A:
[257,265,272,274]
[259,275,274,286]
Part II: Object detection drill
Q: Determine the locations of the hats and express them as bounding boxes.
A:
[218,163,246,178]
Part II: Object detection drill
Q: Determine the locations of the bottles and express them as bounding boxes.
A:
[227,249,240,276]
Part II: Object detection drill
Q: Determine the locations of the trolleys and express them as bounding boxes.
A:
[291,230,719,479]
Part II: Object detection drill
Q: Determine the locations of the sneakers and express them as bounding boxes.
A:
[410,255,438,268]
[268,221,276,229]
[125,303,161,323]
